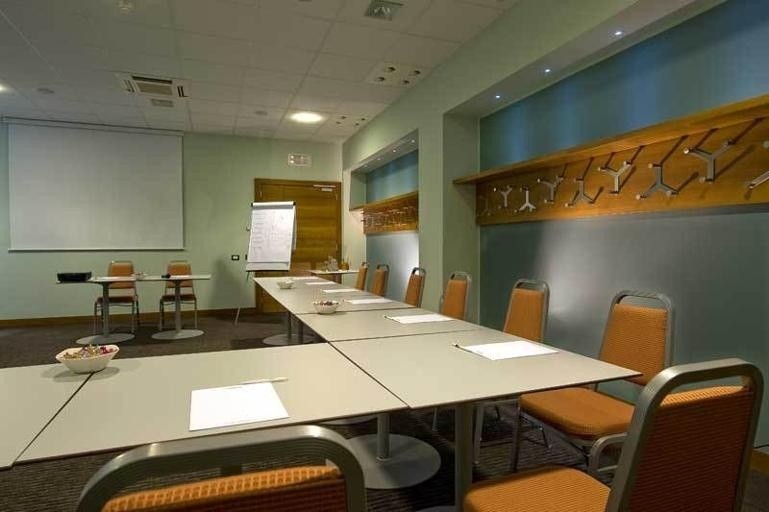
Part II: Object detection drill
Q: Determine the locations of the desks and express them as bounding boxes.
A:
[328,327,644,511]
[306,265,362,286]
[253,276,373,344]
[0,361,99,477]
[13,341,443,492]
[294,306,485,342]
[56,271,138,345]
[134,274,213,341]
[249,343,407,511]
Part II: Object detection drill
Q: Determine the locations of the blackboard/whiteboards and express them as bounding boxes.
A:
[245,200,297,272]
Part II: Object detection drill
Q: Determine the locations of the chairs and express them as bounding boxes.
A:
[459,355,767,510]
[504,285,675,480]
[156,260,201,331]
[92,259,141,336]
[353,262,472,322]
[433,276,551,470]
[72,422,371,509]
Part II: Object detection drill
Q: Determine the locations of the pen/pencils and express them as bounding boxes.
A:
[455,344,473,353]
[240,377,288,384]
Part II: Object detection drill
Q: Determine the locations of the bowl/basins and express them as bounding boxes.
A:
[55,342,120,374]
[277,281,294,288]
[313,300,339,315]
[130,272,149,280]
